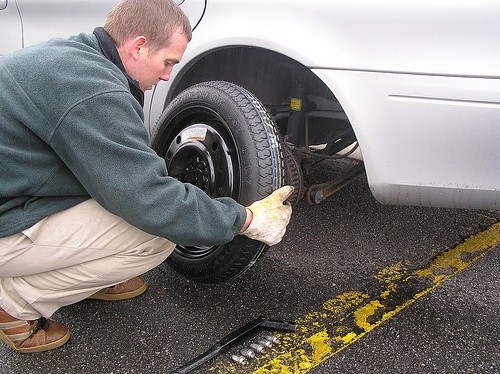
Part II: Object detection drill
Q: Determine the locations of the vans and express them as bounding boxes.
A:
[0,0,500,288]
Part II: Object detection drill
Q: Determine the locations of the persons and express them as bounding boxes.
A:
[0,0,292,348]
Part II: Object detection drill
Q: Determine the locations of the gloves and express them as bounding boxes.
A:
[239,185,295,247]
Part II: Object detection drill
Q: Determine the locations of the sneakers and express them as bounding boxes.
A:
[0,304,71,353]
[87,276,147,300]
